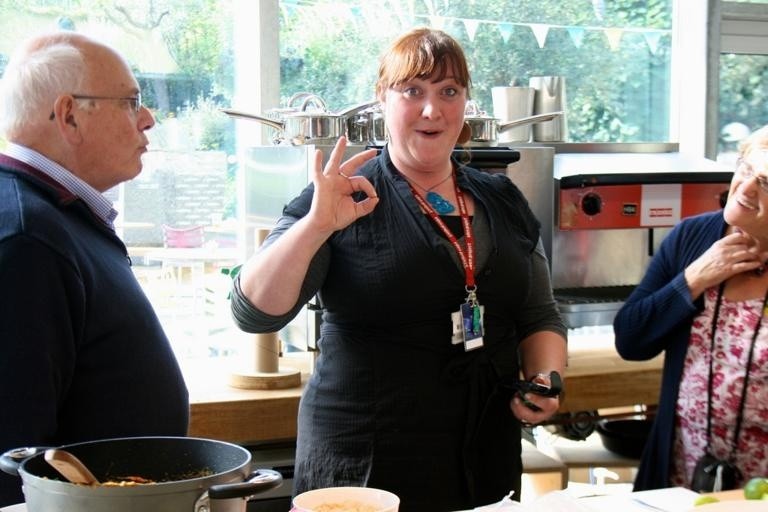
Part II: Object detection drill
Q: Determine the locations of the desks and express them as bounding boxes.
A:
[190,339,666,444]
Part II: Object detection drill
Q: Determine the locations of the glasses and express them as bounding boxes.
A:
[46,88,143,123]
[738,162,767,194]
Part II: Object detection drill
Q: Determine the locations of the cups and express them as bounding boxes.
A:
[290,487,400,512]
[489,85,536,143]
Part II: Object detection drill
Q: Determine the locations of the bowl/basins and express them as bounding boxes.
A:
[596,417,652,459]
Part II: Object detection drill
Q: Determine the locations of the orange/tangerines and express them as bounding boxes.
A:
[744,477,768,500]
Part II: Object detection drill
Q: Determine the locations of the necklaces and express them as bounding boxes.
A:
[734,226,766,276]
[398,172,459,216]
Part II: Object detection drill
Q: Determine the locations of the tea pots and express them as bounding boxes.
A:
[530,74,566,141]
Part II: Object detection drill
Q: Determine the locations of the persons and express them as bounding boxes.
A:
[715,123,750,167]
[0,33,189,511]
[229,28,568,511]
[614,126,767,491]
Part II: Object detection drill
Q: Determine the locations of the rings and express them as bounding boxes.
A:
[339,172,349,183]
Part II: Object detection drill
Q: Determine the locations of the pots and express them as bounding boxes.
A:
[213,91,565,148]
[0,438,283,511]
